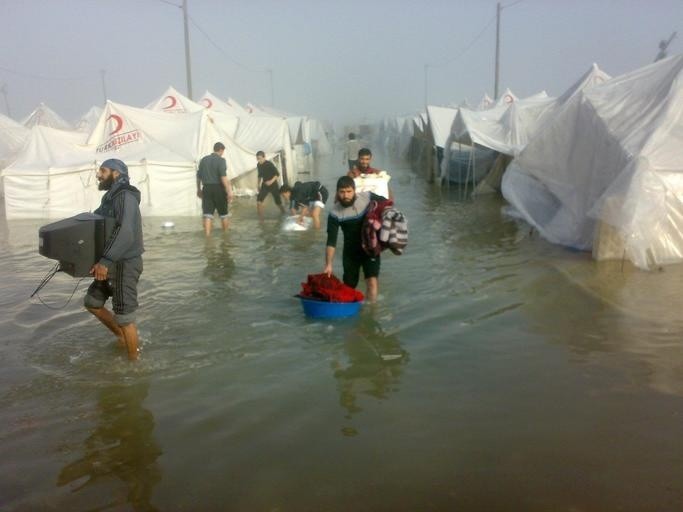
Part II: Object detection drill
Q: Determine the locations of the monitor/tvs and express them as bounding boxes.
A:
[39,211,116,278]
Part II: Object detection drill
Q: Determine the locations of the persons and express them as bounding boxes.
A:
[280,181,329,229]
[346,148,382,178]
[325,176,393,303]
[341,132,362,170]
[84,159,144,362]
[196,142,232,236]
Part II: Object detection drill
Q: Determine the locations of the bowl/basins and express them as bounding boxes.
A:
[292,295,362,318]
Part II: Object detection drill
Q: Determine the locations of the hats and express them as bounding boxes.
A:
[100,157,128,176]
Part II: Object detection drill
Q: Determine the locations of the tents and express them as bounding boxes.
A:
[376,53,682,269]
[1,84,330,221]
